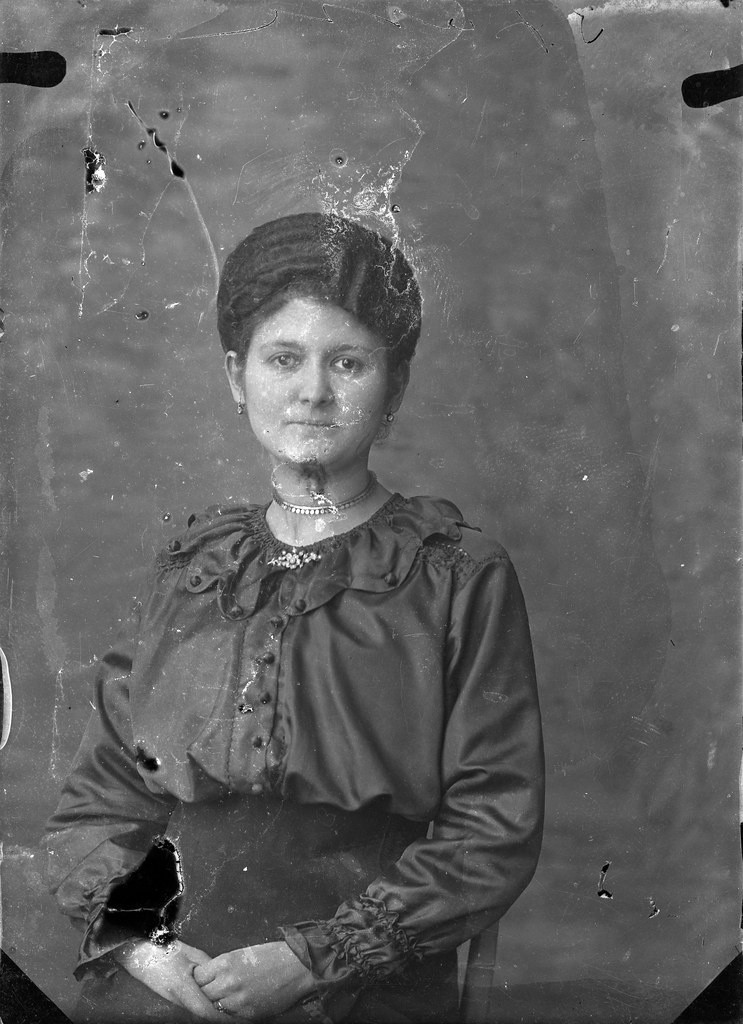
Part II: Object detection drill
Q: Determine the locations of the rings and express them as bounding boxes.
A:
[217,1001,238,1016]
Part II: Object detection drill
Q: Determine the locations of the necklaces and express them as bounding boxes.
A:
[266,471,378,516]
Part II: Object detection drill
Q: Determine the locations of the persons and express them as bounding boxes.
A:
[38,211,547,1024]
